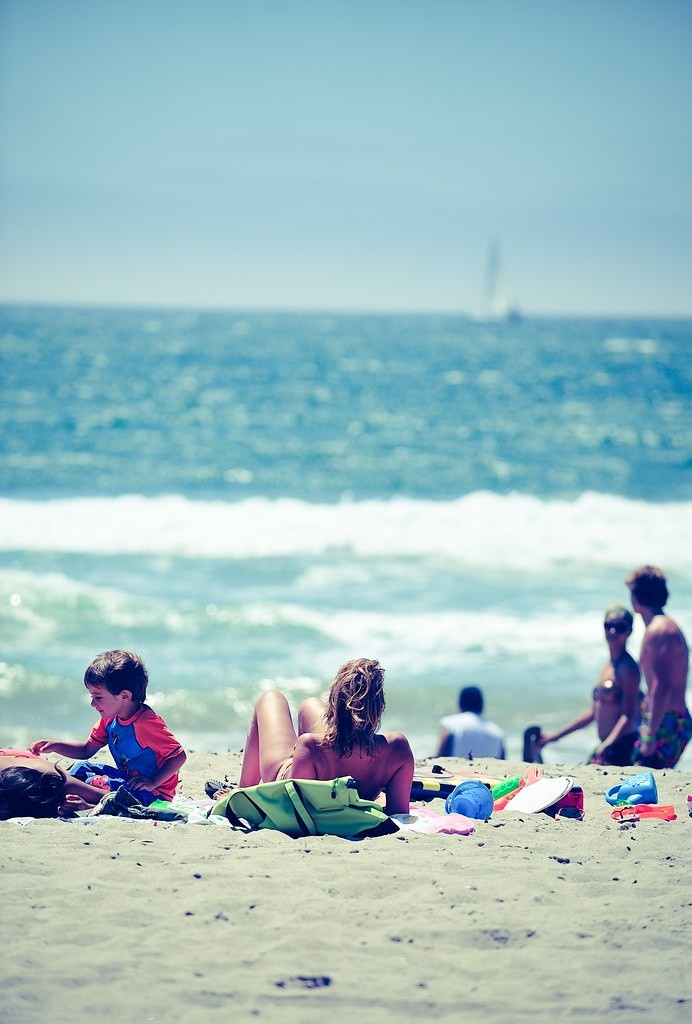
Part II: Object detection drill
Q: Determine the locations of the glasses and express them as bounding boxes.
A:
[603,622,629,634]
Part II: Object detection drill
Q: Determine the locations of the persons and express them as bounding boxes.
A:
[213,657,415,813]
[538,566,692,770]
[0,650,186,822]
[433,688,508,760]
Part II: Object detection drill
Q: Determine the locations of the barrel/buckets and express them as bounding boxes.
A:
[445,779,494,820]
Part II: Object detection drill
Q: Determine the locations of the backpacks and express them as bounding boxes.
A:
[210,775,401,842]
